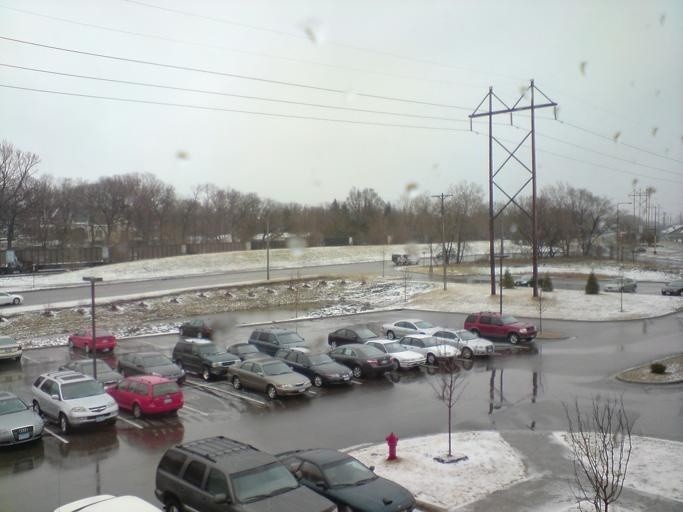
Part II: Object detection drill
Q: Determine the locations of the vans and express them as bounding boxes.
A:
[103,374,183,418]
[32,369,120,434]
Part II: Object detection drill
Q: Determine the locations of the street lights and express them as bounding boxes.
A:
[82,274,105,385]
[244,207,300,281]
[429,193,454,292]
[491,254,509,315]
[616,202,632,261]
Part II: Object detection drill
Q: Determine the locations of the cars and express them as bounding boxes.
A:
[0,290,22,306]
[661,279,682,296]
[52,493,163,512]
[0,390,46,446]
[0,333,22,363]
[636,247,647,253]
[604,276,637,291]
[0,257,37,275]
[513,271,541,288]
[271,447,416,511]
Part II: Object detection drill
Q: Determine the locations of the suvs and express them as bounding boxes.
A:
[391,252,420,265]
[153,434,338,511]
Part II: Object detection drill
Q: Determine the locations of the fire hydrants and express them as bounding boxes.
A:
[385,431,399,460]
